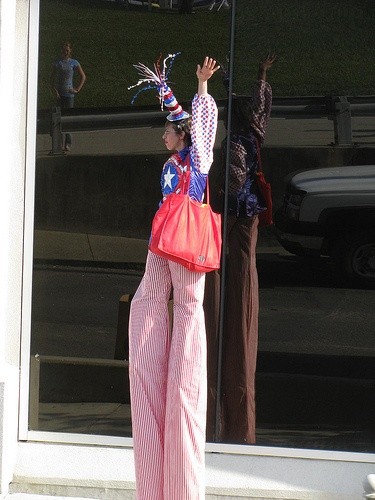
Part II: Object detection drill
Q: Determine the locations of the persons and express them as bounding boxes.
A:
[207,51,277,445]
[127,54,222,500]
[48,38,86,151]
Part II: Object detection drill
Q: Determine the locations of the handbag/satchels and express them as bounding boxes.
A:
[148,193,222,272]
[256,165,274,223]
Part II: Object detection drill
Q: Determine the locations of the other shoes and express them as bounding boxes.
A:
[65,144,71,150]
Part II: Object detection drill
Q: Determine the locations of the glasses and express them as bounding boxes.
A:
[165,130,181,134]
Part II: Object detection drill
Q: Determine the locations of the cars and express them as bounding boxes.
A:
[273,164,375,289]
